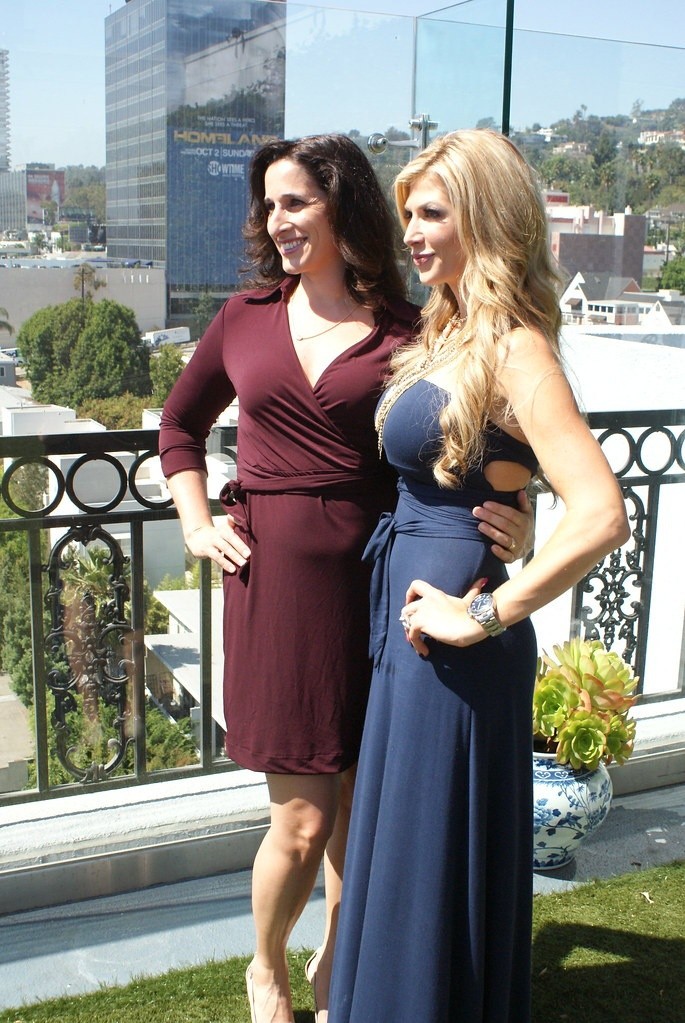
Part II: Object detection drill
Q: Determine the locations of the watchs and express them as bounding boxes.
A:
[469,590,509,639]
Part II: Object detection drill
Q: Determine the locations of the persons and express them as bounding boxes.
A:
[204,0,284,133]
[160,136,537,1023]
[326,129,632,1022]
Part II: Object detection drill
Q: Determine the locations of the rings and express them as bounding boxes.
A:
[507,537,523,554]
[399,611,413,629]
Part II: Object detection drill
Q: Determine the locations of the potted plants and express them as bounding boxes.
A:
[531,636,642,871]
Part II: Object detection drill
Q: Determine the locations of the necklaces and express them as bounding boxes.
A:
[291,295,364,342]
[376,305,478,459]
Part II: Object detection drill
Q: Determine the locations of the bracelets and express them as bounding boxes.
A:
[182,524,220,553]
[525,540,534,550]
